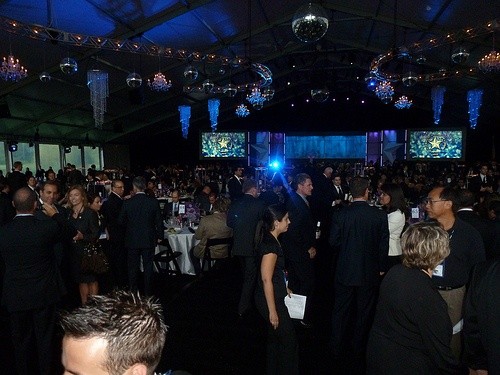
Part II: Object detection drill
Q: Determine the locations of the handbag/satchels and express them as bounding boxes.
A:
[80,244,104,275]
[198,247,216,271]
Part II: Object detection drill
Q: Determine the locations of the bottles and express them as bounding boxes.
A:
[315,221,321,239]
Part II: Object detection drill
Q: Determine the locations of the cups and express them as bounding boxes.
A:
[163,217,195,231]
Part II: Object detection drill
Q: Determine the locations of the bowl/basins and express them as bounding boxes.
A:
[170,227,182,233]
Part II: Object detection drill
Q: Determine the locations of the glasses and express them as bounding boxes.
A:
[115,186,125,189]
[425,198,446,205]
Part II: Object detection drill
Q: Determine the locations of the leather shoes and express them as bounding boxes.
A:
[293,319,312,330]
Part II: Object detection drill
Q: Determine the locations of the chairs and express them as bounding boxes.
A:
[197,236,234,275]
[153,239,182,275]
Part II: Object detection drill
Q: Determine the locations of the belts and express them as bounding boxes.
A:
[436,284,465,292]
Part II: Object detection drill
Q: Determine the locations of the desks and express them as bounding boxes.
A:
[155,226,197,275]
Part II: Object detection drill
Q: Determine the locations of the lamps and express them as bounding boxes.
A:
[291,1,328,44]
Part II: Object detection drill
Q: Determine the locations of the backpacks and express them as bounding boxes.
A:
[238,257,276,327]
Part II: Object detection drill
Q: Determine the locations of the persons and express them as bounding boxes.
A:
[0,161,500,375]
[61,292,167,375]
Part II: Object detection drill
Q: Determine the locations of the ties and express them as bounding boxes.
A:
[338,186,342,195]
[239,178,243,183]
[483,177,485,183]
[210,204,213,211]
[174,204,178,209]
